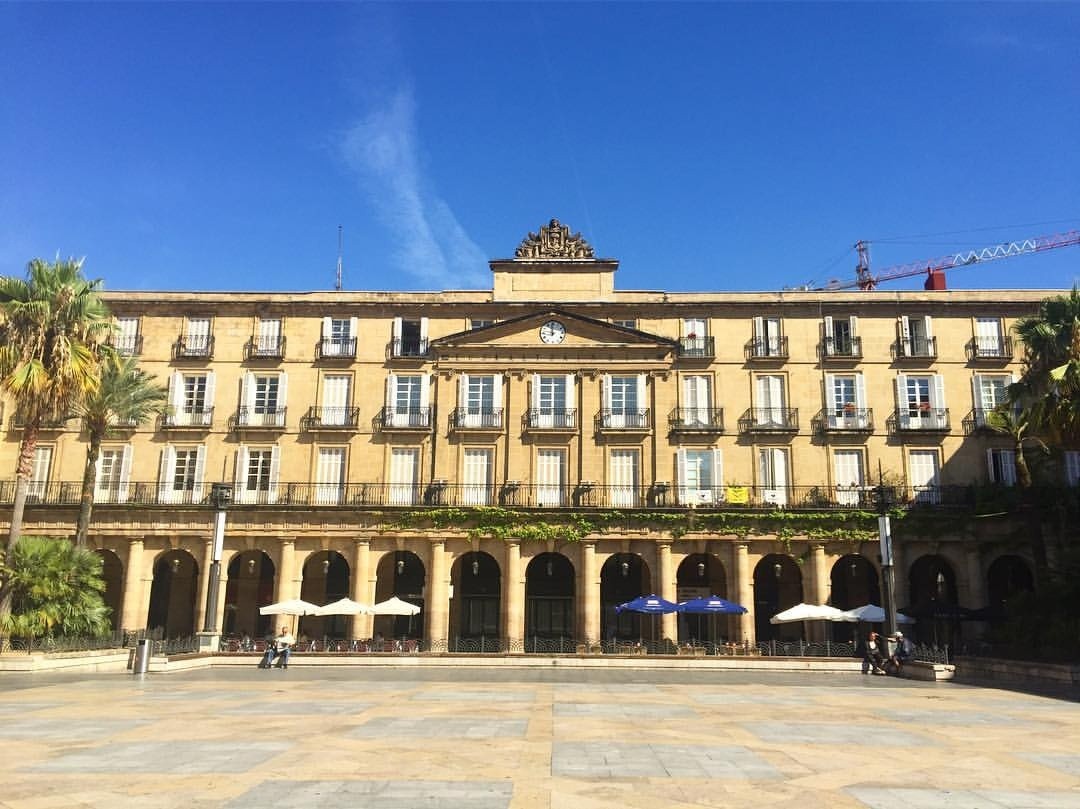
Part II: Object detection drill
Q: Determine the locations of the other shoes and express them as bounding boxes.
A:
[874,668,886,674]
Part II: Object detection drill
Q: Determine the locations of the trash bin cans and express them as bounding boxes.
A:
[134,639,151,672]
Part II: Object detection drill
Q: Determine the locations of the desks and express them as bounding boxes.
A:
[575,643,745,656]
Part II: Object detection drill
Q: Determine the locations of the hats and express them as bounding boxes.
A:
[895,632,904,639]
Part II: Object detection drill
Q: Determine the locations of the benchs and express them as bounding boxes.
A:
[889,657,956,682]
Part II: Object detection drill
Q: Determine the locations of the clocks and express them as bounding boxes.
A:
[540,320,566,345]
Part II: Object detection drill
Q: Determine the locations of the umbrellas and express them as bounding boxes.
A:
[308,597,376,639]
[771,602,917,642]
[616,594,683,641]
[366,597,421,639]
[259,599,319,636]
[683,594,747,642]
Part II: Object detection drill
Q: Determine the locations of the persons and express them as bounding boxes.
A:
[892,632,914,673]
[864,632,887,674]
[265,626,296,669]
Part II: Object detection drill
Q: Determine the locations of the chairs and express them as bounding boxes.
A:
[225,633,419,654]
